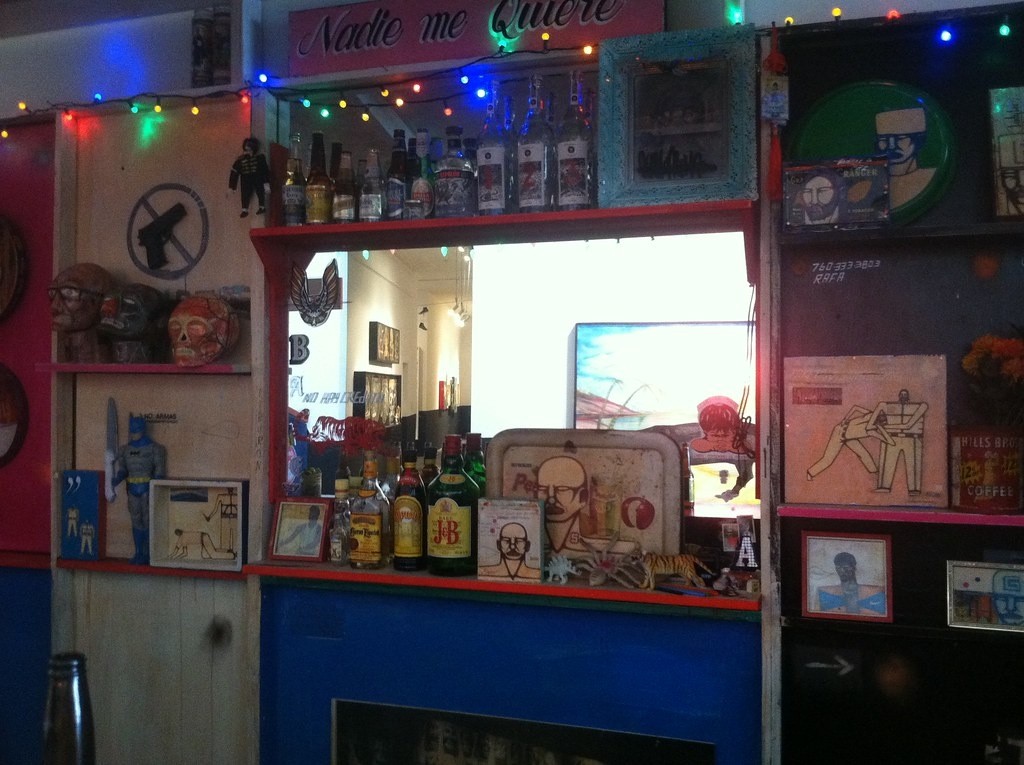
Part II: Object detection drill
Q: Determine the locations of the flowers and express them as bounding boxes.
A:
[961,333,1024,425]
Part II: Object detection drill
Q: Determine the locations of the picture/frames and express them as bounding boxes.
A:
[478,497,545,585]
[947,559,1024,633]
[369,322,400,365]
[486,428,683,581]
[268,496,334,563]
[783,356,949,509]
[597,25,759,210]
[781,155,891,231]
[800,530,893,623]
[353,372,402,426]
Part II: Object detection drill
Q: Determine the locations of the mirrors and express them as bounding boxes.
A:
[281,229,762,520]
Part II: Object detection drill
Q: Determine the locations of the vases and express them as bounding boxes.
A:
[948,424,1024,515]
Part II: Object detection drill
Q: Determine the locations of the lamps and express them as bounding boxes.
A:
[447,246,470,328]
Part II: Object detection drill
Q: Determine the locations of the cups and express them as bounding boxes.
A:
[590,492,622,540]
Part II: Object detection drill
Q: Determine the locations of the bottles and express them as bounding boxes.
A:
[383,438,440,572]
[329,142,385,223]
[557,72,595,210]
[326,450,392,568]
[426,433,486,577]
[434,124,478,218]
[42,651,95,765]
[476,82,517,217]
[278,131,332,226]
[385,129,442,220]
[517,73,556,213]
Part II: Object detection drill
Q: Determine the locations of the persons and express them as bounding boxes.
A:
[278,505,323,554]
[46,264,115,363]
[97,283,172,364]
[168,297,240,367]
[107,414,168,563]
[227,135,273,218]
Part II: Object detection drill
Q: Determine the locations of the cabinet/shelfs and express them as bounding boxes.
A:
[1,0,1024,765]
